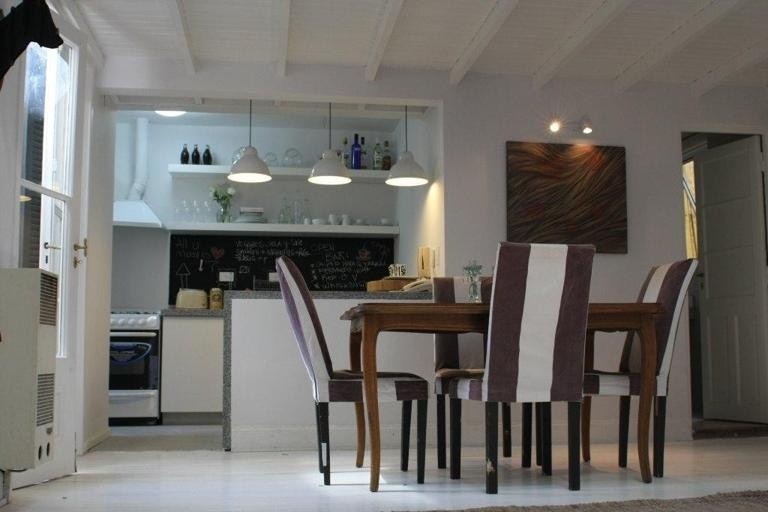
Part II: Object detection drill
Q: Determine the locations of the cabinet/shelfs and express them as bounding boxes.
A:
[167,222,401,233]
[161,307,223,426]
[167,163,390,178]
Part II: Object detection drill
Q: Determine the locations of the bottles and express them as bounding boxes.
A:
[374,139,383,170]
[352,133,361,170]
[341,137,352,170]
[192,145,199,164]
[361,138,368,169]
[203,145,212,164]
[181,144,188,164]
[383,140,391,169]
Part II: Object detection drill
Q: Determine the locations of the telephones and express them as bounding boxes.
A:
[389,279,432,293]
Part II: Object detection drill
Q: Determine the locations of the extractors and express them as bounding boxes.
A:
[105,198,163,228]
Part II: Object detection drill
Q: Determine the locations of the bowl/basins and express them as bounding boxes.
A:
[312,219,321,224]
[175,288,209,310]
[380,218,392,225]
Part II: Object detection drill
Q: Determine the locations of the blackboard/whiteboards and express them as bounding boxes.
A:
[169,232,396,306]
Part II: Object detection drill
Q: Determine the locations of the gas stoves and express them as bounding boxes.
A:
[108,309,159,330]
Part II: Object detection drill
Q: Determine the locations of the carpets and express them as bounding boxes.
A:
[388,490,767,512]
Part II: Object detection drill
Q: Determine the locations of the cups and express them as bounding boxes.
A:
[329,214,342,224]
[176,200,213,223]
[342,214,350,225]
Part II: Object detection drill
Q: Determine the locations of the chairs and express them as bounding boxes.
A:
[277,256,429,486]
[448,242,595,495]
[582,258,699,479]
[434,277,532,469]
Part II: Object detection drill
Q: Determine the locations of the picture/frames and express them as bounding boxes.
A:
[505,140,628,254]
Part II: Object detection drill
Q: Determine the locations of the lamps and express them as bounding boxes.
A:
[385,106,430,187]
[227,100,273,184]
[308,103,352,186]
